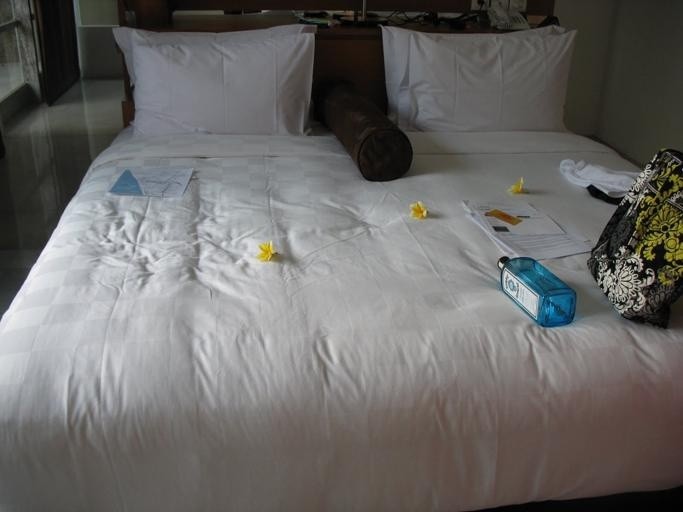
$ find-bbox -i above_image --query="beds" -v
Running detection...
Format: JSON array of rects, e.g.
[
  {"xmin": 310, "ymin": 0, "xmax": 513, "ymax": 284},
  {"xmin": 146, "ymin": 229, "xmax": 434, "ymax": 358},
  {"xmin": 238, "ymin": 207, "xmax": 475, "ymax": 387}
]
[{"xmin": 1, "ymin": 0, "xmax": 683, "ymax": 511}]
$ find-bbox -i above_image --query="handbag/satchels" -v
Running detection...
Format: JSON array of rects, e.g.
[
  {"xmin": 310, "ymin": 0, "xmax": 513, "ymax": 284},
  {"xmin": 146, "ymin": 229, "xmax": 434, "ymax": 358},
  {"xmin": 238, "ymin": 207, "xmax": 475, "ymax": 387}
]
[{"xmin": 586, "ymin": 148, "xmax": 681, "ymax": 328}]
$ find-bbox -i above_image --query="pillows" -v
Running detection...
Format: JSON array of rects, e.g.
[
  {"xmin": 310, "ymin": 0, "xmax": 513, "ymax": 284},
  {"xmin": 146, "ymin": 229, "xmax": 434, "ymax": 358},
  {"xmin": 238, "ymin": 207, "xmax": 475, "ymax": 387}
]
[
  {"xmin": 377, "ymin": 22, "xmax": 578, "ymax": 132},
  {"xmin": 111, "ymin": 23, "xmax": 319, "ymax": 137},
  {"xmin": 312, "ymin": 84, "xmax": 413, "ymax": 182}
]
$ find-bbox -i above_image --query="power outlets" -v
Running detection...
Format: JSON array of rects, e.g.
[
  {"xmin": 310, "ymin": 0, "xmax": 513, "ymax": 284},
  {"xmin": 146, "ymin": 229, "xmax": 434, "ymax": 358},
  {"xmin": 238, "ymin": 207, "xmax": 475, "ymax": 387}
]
[{"xmin": 471, "ymin": 0, "xmax": 490, "ymax": 11}]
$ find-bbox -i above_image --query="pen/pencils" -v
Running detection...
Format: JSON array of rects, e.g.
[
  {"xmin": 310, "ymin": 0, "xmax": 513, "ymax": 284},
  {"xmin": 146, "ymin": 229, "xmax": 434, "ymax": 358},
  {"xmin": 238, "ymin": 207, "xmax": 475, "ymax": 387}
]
[{"xmin": 517, "ymin": 216, "xmax": 546, "ymax": 218}]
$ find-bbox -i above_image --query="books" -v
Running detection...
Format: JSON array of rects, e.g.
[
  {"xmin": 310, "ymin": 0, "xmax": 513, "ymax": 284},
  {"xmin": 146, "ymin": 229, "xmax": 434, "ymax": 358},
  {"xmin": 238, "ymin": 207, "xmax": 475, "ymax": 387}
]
[{"xmin": 461, "ymin": 199, "xmax": 593, "ymax": 259}]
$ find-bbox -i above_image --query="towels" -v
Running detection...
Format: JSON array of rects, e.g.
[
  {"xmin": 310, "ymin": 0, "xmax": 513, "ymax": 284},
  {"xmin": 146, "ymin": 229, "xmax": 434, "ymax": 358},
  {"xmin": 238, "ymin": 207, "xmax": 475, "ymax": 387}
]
[{"xmin": 560, "ymin": 154, "xmax": 642, "ymax": 198}]
